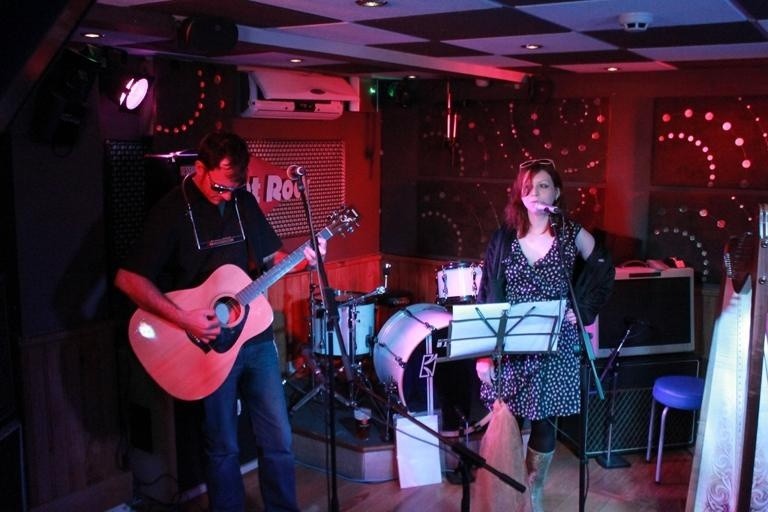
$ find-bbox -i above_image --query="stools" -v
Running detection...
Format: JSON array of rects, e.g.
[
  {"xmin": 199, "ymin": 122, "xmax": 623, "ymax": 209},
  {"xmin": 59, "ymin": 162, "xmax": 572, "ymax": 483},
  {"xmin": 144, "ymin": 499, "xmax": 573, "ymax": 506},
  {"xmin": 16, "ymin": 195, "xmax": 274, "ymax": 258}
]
[{"xmin": 644, "ymin": 374, "xmax": 704, "ymax": 482}]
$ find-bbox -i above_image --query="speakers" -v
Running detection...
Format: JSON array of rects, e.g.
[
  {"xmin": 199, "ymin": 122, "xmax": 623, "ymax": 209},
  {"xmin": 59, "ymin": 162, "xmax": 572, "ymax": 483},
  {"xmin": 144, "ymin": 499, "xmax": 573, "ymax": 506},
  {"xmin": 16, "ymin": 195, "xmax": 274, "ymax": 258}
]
[
  {"xmin": 555, "ymin": 355, "xmax": 699, "ymax": 458},
  {"xmin": 584, "ymin": 260, "xmax": 695, "ymax": 360}
]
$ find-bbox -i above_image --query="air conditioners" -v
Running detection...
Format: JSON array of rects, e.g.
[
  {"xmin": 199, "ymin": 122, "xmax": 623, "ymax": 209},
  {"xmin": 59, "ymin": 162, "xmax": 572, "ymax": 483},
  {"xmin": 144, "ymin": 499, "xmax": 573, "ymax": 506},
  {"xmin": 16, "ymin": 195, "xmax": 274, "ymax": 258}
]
[{"xmin": 239, "ymin": 65, "xmax": 361, "ymax": 121}]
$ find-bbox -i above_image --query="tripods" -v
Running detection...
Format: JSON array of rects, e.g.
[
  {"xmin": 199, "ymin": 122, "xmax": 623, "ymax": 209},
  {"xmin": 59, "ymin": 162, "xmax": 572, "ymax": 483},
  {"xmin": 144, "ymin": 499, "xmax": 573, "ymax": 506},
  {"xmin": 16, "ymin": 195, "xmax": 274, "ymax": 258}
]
[{"xmin": 290, "ymin": 270, "xmax": 395, "ymax": 441}]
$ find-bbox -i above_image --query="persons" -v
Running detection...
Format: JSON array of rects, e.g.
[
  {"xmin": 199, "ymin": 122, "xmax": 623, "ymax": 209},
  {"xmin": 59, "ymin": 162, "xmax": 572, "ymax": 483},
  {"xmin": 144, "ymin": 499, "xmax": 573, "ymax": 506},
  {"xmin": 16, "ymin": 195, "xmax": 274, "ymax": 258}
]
[
  {"xmin": 112, "ymin": 124, "xmax": 327, "ymax": 512},
  {"xmin": 469, "ymin": 157, "xmax": 618, "ymax": 512}
]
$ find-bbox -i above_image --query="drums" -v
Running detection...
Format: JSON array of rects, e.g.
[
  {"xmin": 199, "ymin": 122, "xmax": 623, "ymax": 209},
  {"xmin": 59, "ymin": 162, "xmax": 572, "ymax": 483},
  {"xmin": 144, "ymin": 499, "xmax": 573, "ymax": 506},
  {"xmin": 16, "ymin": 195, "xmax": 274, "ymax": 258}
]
[
  {"xmin": 308, "ymin": 290, "xmax": 379, "ymax": 360},
  {"xmin": 372, "ymin": 303, "xmax": 494, "ymax": 438},
  {"xmin": 435, "ymin": 260, "xmax": 485, "ymax": 305}
]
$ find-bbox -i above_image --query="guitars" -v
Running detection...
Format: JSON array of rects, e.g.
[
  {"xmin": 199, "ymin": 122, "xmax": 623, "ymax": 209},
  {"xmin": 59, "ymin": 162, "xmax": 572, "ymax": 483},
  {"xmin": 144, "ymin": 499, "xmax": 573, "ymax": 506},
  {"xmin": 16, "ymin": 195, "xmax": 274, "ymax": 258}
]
[{"xmin": 127, "ymin": 205, "xmax": 362, "ymax": 401}]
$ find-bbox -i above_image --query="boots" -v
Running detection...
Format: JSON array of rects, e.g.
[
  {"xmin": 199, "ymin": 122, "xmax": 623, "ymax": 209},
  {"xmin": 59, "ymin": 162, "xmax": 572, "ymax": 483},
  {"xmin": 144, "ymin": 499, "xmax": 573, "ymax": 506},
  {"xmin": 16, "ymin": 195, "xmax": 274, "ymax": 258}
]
[{"xmin": 522, "ymin": 444, "xmax": 558, "ymax": 511}]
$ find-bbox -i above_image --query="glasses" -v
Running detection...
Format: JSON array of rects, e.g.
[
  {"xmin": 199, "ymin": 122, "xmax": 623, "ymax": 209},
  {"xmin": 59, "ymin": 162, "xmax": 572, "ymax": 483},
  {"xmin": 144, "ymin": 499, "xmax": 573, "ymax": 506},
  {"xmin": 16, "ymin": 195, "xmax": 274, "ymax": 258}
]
[
  {"xmin": 206, "ymin": 170, "xmax": 247, "ymax": 195},
  {"xmin": 519, "ymin": 159, "xmax": 556, "ymax": 173}
]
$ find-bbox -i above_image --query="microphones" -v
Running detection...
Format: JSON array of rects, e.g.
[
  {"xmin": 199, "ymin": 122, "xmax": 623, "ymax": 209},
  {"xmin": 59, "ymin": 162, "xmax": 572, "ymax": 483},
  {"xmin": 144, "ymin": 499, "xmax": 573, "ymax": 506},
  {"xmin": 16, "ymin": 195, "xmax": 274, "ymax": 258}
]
[
  {"xmin": 536, "ymin": 202, "xmax": 558, "ymax": 214},
  {"xmin": 287, "ymin": 165, "xmax": 305, "ymax": 180}
]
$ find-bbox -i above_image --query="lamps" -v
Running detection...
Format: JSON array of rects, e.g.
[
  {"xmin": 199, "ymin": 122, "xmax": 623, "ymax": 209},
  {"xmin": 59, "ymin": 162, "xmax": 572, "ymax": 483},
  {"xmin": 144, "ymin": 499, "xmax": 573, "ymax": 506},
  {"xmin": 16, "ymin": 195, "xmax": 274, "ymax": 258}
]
[{"xmin": 65, "ymin": 47, "xmax": 154, "ymax": 115}]
